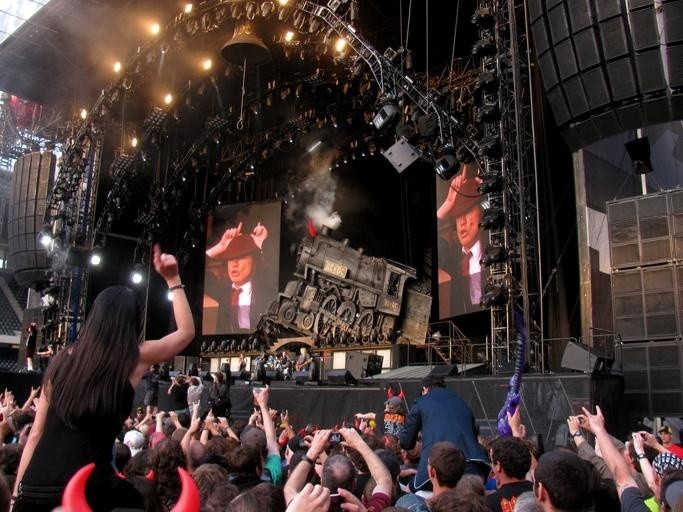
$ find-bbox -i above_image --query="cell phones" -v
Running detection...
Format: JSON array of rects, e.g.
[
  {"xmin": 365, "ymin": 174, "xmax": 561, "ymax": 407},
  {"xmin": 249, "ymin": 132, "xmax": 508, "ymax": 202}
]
[
  {"xmin": 640, "ymin": 434, "xmax": 646, "ymax": 441},
  {"xmin": 252, "ymin": 381, "xmax": 265, "ymax": 410},
  {"xmin": 331, "ymin": 495, "xmax": 345, "ymax": 511},
  {"xmin": 328, "ymin": 432, "xmax": 342, "ymax": 445}
]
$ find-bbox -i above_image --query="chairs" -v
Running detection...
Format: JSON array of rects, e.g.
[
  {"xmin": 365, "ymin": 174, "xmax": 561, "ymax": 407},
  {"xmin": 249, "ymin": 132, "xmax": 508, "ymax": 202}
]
[{"xmin": 0, "ymin": 274, "xmax": 27, "ymax": 373}]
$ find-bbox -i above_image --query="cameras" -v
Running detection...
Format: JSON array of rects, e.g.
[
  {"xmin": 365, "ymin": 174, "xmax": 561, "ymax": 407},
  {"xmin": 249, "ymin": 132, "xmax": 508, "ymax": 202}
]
[{"xmin": 208, "ymin": 395, "xmax": 232, "ymax": 418}]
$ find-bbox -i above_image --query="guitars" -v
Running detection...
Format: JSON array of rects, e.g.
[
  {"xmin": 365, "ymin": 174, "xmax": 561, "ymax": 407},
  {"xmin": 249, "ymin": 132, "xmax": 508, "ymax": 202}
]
[{"xmin": 295, "ymin": 357, "xmax": 314, "ymax": 372}]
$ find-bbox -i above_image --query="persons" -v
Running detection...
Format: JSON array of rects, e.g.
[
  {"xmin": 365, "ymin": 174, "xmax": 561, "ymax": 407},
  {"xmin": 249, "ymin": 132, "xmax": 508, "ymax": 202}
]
[
  {"xmin": 6, "ymin": 245, "xmax": 196, "ymax": 511},
  {"xmin": 204, "ymin": 220, "xmax": 278, "ymax": 335},
  {"xmin": 434, "ymin": 163, "xmax": 495, "ymax": 318},
  {"xmin": 0, "ymin": 344, "xmax": 682, "ymax": 512}
]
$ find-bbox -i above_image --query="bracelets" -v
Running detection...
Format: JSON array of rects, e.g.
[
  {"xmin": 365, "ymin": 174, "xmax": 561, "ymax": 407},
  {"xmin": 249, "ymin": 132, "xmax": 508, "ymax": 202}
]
[{"xmin": 168, "ymin": 284, "xmax": 187, "ymax": 294}]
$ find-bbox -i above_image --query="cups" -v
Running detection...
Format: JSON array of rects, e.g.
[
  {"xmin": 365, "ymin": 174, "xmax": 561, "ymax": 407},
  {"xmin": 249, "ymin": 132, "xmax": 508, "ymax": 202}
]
[{"xmin": 253, "ymin": 386, "xmax": 264, "ymax": 405}]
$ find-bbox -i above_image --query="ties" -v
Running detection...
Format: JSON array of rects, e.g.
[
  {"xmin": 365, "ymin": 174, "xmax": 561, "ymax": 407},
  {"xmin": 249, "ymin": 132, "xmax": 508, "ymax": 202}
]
[
  {"xmin": 230, "ymin": 288, "xmax": 242, "ymax": 329},
  {"xmin": 462, "ymin": 252, "xmax": 472, "ymax": 280}
]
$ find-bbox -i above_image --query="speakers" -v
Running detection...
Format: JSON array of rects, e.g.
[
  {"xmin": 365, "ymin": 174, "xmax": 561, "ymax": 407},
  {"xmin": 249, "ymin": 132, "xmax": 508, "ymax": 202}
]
[
  {"xmin": 610, "ymin": 263, "xmax": 679, "ymax": 344},
  {"xmin": 231, "ymin": 371, "xmax": 243, "ymax": 385},
  {"xmin": 266, "ymin": 371, "xmax": 281, "ymax": 384},
  {"xmin": 616, "ymin": 341, "xmax": 682, "ymax": 417},
  {"xmin": 669, "ymin": 188, "xmax": 683, "ymax": 263},
  {"xmin": 327, "ymin": 370, "xmax": 356, "ymax": 385},
  {"xmin": 430, "ymin": 365, "xmax": 458, "ymax": 377},
  {"xmin": 292, "ymin": 371, "xmax": 313, "ymax": 385},
  {"xmin": 674, "ymin": 262, "xmax": 683, "ymax": 340},
  {"xmin": 200, "ymin": 371, "xmax": 214, "ymax": 381},
  {"xmin": 605, "ymin": 193, "xmax": 674, "ymax": 270}
]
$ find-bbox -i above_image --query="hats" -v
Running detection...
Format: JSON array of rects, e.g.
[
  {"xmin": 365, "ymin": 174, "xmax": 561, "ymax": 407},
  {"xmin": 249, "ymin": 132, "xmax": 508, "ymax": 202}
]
[
  {"xmin": 659, "ymin": 426, "xmax": 672, "ymax": 433},
  {"xmin": 448, "ymin": 181, "xmax": 484, "ymax": 216},
  {"xmin": 222, "ymin": 237, "xmax": 259, "ymax": 259},
  {"xmin": 124, "ymin": 431, "xmax": 145, "ymax": 458}
]
